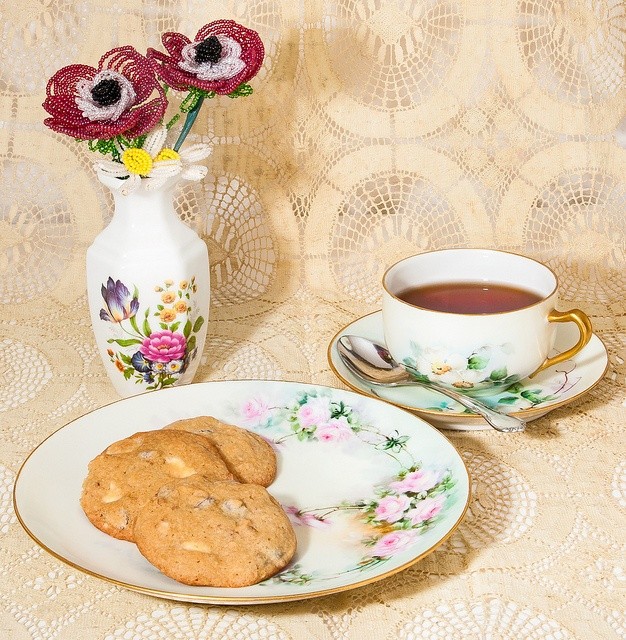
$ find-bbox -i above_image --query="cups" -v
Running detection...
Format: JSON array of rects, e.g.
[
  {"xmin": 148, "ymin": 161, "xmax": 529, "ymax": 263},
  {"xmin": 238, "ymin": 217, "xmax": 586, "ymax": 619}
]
[{"xmin": 381, "ymin": 247, "xmax": 594, "ymax": 390}]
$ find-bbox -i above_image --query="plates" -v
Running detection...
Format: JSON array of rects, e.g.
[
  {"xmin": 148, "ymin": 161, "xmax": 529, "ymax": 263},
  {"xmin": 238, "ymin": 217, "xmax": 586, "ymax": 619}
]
[
  {"xmin": 13, "ymin": 380, "xmax": 473, "ymax": 605},
  {"xmin": 328, "ymin": 308, "xmax": 611, "ymax": 430}
]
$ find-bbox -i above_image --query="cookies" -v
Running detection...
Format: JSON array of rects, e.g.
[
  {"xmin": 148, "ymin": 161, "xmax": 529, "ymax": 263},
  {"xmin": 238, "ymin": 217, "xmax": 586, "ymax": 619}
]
[
  {"xmin": 134, "ymin": 476, "xmax": 296, "ymax": 587},
  {"xmin": 83, "ymin": 431, "xmax": 243, "ymax": 543},
  {"xmin": 160, "ymin": 416, "xmax": 277, "ymax": 487}
]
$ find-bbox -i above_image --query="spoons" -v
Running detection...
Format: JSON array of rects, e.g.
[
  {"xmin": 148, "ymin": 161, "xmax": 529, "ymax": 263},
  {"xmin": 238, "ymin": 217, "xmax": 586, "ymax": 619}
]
[{"xmin": 336, "ymin": 335, "xmax": 527, "ymax": 434}]
